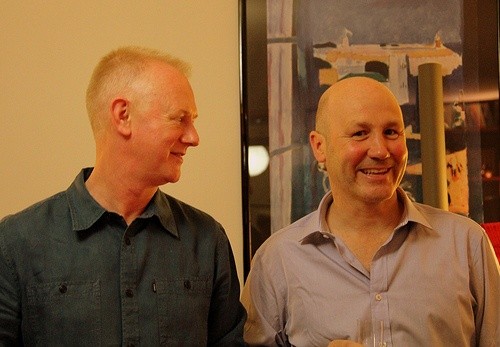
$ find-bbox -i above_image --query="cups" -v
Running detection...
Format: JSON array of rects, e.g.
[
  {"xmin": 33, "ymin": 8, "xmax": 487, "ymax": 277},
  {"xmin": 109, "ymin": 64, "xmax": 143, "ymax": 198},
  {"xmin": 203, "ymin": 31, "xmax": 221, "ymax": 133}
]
[{"xmin": 356, "ymin": 319, "xmax": 384, "ymax": 347}]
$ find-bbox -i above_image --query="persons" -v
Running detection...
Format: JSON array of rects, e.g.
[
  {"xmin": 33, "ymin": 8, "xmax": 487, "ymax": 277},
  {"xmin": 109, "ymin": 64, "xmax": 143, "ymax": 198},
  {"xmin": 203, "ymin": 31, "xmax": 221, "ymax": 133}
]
[
  {"xmin": 243, "ymin": 77, "xmax": 500, "ymax": 347},
  {"xmin": 0, "ymin": 47, "xmax": 247, "ymax": 347}
]
